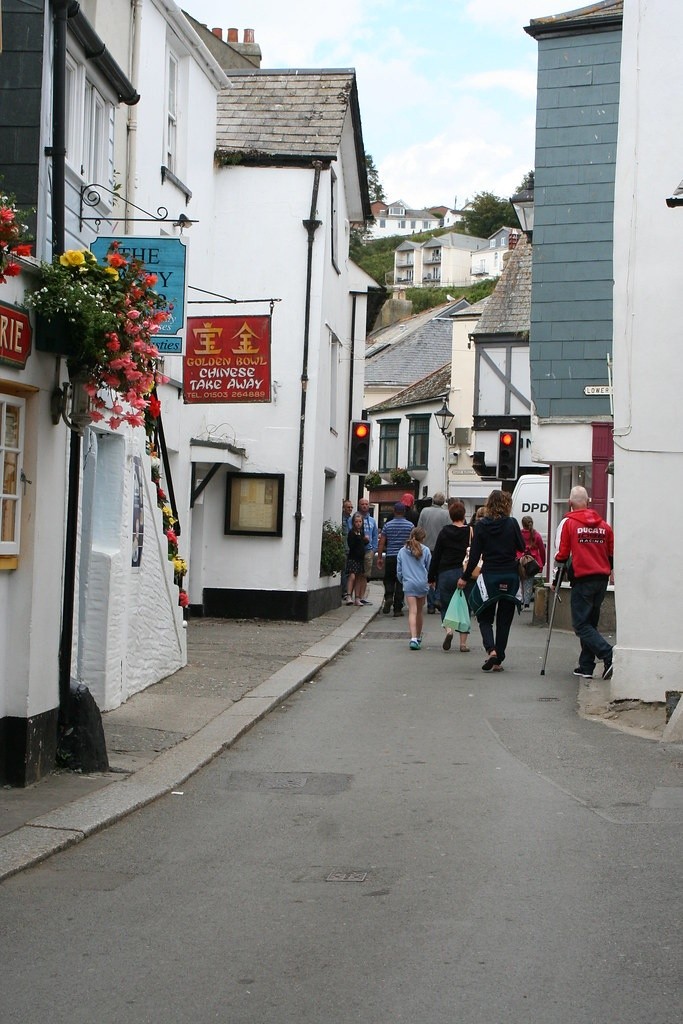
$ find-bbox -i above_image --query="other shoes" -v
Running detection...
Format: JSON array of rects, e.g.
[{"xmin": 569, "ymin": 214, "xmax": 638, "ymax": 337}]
[
  {"xmin": 602, "ymin": 646, "xmax": 613, "ymax": 679},
  {"xmin": 427, "ymin": 608, "xmax": 435, "ymax": 614},
  {"xmin": 354, "ymin": 601, "xmax": 363, "ymax": 606},
  {"xmin": 573, "ymin": 667, "xmax": 593, "ymax": 678},
  {"xmin": 383, "ymin": 598, "xmax": 393, "ymax": 613},
  {"xmin": 408, "ymin": 640, "xmax": 421, "ymax": 649},
  {"xmin": 346, "ymin": 601, "xmax": 352, "ymax": 606},
  {"xmin": 460, "ymin": 646, "xmax": 469, "ymax": 652},
  {"xmin": 443, "ymin": 632, "xmax": 453, "ymax": 650},
  {"xmin": 360, "ymin": 599, "xmax": 371, "ymax": 605},
  {"xmin": 484, "ymin": 659, "xmax": 503, "ymax": 671},
  {"xmin": 393, "ymin": 611, "xmax": 404, "ymax": 617},
  {"xmin": 481, "ymin": 655, "xmax": 500, "ymax": 670}
]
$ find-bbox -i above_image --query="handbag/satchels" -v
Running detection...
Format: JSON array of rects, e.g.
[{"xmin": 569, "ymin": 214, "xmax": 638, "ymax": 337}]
[{"xmin": 441, "ymin": 587, "xmax": 470, "ymax": 633}]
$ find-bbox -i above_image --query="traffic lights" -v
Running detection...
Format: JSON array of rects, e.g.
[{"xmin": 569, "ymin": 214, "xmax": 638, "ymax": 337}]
[
  {"xmin": 494, "ymin": 428, "xmax": 520, "ymax": 481},
  {"xmin": 348, "ymin": 420, "xmax": 372, "ymax": 476}
]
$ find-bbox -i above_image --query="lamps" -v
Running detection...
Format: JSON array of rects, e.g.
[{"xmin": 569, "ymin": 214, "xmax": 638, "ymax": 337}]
[
  {"xmin": 173, "ymin": 214, "xmax": 193, "ymax": 229},
  {"xmin": 434, "ymin": 398, "xmax": 455, "ymax": 440}
]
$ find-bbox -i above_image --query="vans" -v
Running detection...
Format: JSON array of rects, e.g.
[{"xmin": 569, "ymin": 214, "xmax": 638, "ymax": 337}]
[{"xmin": 509, "ymin": 474, "xmax": 549, "ymax": 550}]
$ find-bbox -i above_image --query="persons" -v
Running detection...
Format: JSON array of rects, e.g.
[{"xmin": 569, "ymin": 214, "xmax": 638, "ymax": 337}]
[
  {"xmin": 375, "ymin": 490, "xmax": 485, "ymax": 615},
  {"xmin": 554, "ymin": 486, "xmax": 616, "ymax": 679},
  {"xmin": 456, "ymin": 490, "xmax": 528, "ymax": 672},
  {"xmin": 428, "ymin": 501, "xmax": 471, "ymax": 651},
  {"xmin": 516, "ymin": 515, "xmax": 546, "ymax": 612},
  {"xmin": 343, "ymin": 496, "xmax": 379, "ymax": 605},
  {"xmin": 395, "ymin": 526, "xmax": 431, "ymax": 651}
]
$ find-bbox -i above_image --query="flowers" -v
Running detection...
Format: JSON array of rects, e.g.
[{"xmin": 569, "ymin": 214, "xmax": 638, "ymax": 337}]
[
  {"xmin": 0, "ymin": 173, "xmax": 34, "ymax": 284},
  {"xmin": 21, "ymin": 241, "xmax": 189, "ymax": 610}
]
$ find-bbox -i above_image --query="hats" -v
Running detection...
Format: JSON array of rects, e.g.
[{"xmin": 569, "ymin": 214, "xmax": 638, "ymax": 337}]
[{"xmin": 394, "ymin": 502, "xmax": 405, "ymax": 512}]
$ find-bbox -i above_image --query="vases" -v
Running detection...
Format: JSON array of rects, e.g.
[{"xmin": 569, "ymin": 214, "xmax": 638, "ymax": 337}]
[{"xmin": 34, "ymin": 309, "xmax": 86, "ymax": 359}]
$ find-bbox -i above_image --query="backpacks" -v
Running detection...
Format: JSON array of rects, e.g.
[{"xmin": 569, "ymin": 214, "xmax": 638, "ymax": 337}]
[{"xmin": 518, "ymin": 551, "xmax": 540, "ymax": 578}]
